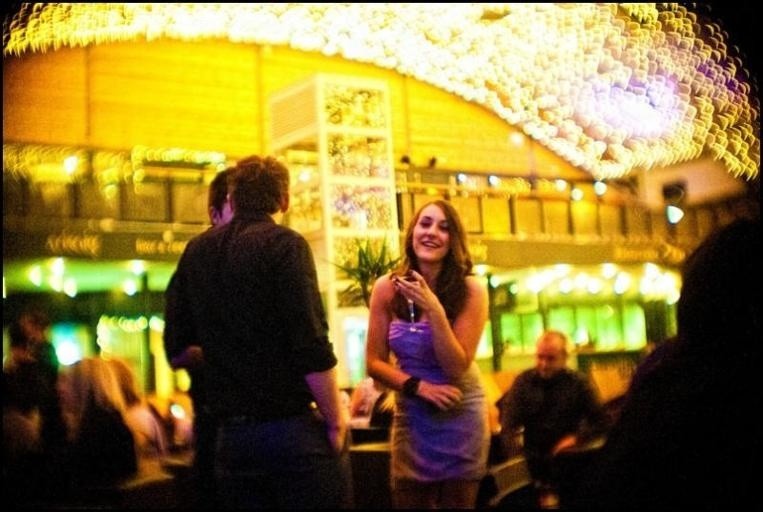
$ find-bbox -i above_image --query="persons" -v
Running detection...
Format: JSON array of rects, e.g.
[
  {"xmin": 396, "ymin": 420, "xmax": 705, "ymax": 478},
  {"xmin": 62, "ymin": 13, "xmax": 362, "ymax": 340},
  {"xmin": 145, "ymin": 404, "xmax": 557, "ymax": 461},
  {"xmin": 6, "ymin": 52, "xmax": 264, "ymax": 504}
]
[
  {"xmin": 179, "ymin": 154, "xmax": 352, "ymax": 511},
  {"xmin": 158, "ymin": 167, "xmax": 241, "ymax": 512},
  {"xmin": 497, "ymin": 215, "xmax": 763, "ymax": 511},
  {"xmin": 0, "ymin": 296, "xmax": 171, "ymax": 510},
  {"xmin": 355, "ymin": 371, "xmax": 394, "ymax": 426},
  {"xmin": 365, "ymin": 199, "xmax": 494, "ymax": 510}
]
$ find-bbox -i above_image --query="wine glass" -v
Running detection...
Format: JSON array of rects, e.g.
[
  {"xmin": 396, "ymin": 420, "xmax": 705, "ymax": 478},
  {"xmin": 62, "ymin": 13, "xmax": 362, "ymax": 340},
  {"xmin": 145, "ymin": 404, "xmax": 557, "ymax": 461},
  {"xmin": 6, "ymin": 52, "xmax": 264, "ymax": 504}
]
[{"xmin": 400, "ymin": 275, "xmax": 424, "ymax": 334}]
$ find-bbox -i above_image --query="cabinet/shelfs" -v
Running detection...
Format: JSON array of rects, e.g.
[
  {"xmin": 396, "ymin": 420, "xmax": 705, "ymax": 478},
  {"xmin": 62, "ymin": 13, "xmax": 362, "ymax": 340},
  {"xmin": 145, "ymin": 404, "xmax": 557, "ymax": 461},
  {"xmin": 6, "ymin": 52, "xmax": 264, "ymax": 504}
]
[{"xmin": 264, "ymin": 77, "xmax": 404, "ymax": 389}]
